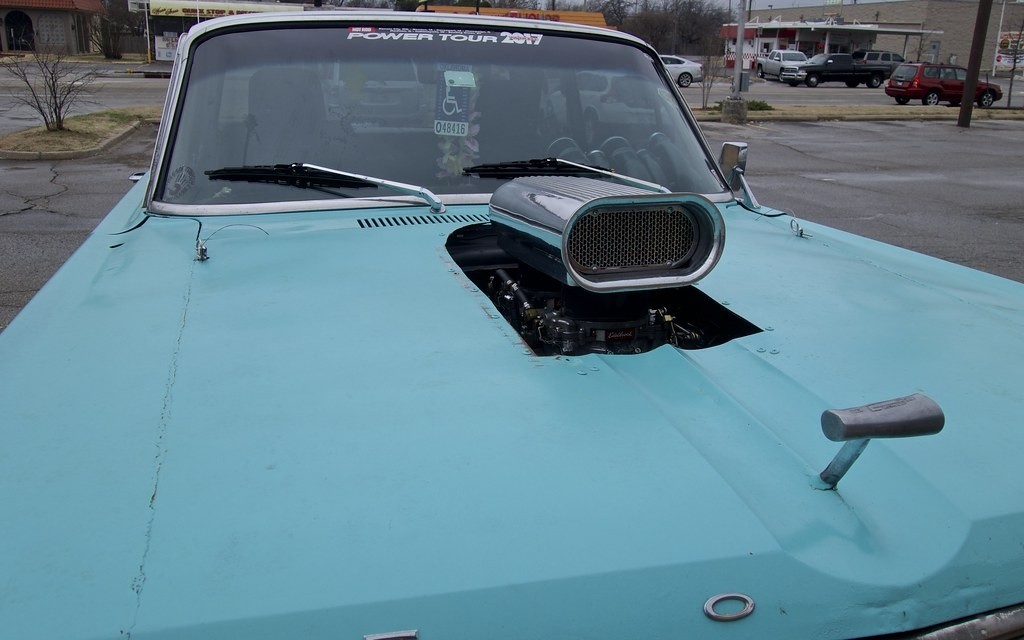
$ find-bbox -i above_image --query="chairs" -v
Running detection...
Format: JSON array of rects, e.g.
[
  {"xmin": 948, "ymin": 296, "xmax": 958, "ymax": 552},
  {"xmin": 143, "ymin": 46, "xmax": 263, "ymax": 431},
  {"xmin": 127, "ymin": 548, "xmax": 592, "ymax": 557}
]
[
  {"xmin": 465, "ymin": 81, "xmax": 574, "ymax": 176},
  {"xmin": 191, "ymin": 69, "xmax": 372, "ymax": 185}
]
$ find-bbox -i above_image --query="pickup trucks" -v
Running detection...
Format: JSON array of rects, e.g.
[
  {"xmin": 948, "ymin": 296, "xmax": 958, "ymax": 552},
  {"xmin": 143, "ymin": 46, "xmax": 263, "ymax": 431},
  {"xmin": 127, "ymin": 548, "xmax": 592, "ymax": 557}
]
[
  {"xmin": 755, "ymin": 49, "xmax": 809, "ymax": 84},
  {"xmin": 779, "ymin": 53, "xmax": 892, "ymax": 89}
]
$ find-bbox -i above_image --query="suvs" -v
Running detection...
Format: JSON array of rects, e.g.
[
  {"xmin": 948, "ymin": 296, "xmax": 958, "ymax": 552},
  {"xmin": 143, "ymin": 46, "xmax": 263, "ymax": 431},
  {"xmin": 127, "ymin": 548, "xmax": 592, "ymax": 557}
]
[
  {"xmin": 852, "ymin": 48, "xmax": 905, "ymax": 80},
  {"xmin": 883, "ymin": 61, "xmax": 1004, "ymax": 109}
]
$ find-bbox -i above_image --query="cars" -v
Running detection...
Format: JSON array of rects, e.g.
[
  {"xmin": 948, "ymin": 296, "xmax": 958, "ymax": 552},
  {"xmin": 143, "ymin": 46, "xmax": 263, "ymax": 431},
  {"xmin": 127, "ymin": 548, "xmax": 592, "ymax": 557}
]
[
  {"xmin": 1, "ymin": 10, "xmax": 1024, "ymax": 639},
  {"xmin": 660, "ymin": 54, "xmax": 706, "ymax": 88}
]
